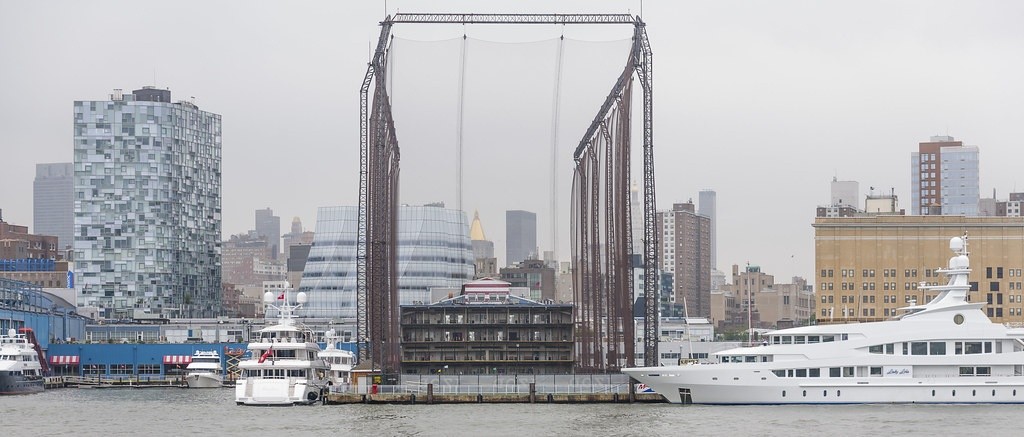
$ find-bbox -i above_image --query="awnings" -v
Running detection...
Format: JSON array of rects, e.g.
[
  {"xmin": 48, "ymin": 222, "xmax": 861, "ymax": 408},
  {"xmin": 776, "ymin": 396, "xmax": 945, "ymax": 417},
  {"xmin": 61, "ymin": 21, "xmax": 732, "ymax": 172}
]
[
  {"xmin": 50, "ymin": 356, "xmax": 80, "ymax": 364},
  {"xmin": 163, "ymin": 355, "xmax": 192, "ymax": 364}
]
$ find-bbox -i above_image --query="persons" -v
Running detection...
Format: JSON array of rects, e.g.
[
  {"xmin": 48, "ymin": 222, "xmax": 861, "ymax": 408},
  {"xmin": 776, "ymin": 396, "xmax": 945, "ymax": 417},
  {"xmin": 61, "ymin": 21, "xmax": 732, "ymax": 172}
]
[{"xmin": 762, "ymin": 337, "xmax": 766, "ymax": 342}]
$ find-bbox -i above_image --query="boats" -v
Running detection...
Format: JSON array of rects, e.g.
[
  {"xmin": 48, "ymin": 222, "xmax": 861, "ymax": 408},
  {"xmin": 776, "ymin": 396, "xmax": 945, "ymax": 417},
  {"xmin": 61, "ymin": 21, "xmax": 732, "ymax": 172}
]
[
  {"xmin": 620, "ymin": 230, "xmax": 1024, "ymax": 404},
  {"xmin": 185, "ymin": 350, "xmax": 223, "ymax": 387},
  {"xmin": 319, "ymin": 328, "xmax": 355, "ymax": 393},
  {"xmin": 234, "ymin": 277, "xmax": 331, "ymax": 406},
  {"xmin": 0, "ymin": 329, "xmax": 45, "ymax": 395}
]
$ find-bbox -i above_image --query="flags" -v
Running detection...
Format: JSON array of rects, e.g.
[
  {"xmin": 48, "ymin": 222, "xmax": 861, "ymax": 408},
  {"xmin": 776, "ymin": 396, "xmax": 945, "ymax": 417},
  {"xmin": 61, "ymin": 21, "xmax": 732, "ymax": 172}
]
[
  {"xmin": 278, "ymin": 294, "xmax": 284, "ymax": 300},
  {"xmin": 258, "ymin": 347, "xmax": 272, "ymax": 363}
]
